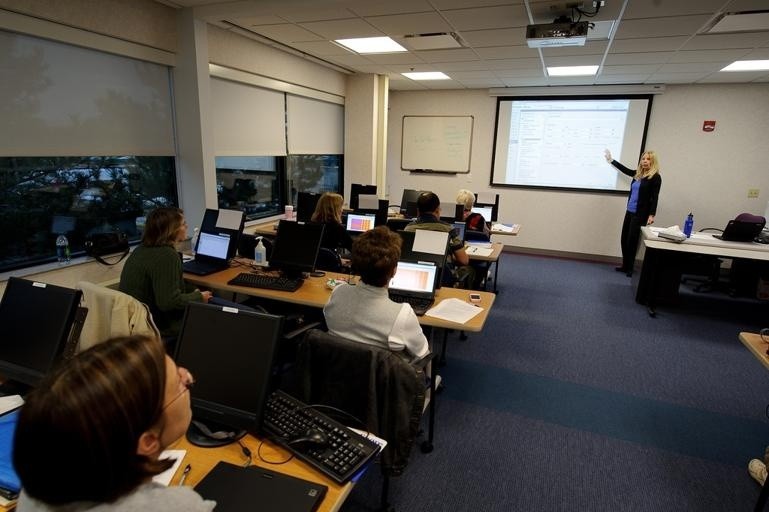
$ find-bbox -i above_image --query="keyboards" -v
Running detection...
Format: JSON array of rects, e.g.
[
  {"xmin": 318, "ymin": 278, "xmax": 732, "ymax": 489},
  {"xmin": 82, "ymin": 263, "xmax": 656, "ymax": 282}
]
[
  {"xmin": 253, "ymin": 387, "xmax": 382, "ymax": 485},
  {"xmin": 226, "ymin": 271, "xmax": 305, "ymax": 292}
]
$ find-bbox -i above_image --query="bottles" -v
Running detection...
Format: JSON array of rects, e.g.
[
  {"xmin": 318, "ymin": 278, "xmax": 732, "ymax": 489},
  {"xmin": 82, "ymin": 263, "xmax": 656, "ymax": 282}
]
[
  {"xmin": 285, "ymin": 205, "xmax": 294, "ymax": 220},
  {"xmin": 55, "ymin": 232, "xmax": 72, "ymax": 265},
  {"xmin": 682, "ymin": 213, "xmax": 694, "ymax": 238}
]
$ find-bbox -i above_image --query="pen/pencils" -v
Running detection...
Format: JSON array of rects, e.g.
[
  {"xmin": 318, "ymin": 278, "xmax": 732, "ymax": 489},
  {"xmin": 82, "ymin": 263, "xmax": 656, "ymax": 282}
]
[
  {"xmin": 178, "ymin": 464, "xmax": 192, "ymax": 486},
  {"xmin": 473, "ymin": 248, "xmax": 478, "ymax": 252}
]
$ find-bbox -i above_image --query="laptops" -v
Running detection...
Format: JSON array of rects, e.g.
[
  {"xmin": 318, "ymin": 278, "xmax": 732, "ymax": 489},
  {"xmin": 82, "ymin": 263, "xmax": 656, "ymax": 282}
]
[
  {"xmin": 343, "ymin": 211, "xmax": 379, "ymax": 238},
  {"xmin": 49, "ymin": 214, "xmax": 78, "ymax": 235},
  {"xmin": 713, "ymin": 219, "xmax": 763, "ymax": 244},
  {"xmin": 387, "ymin": 259, "xmax": 438, "ymax": 316},
  {"xmin": 448, "ymin": 220, "xmax": 467, "ymax": 242},
  {"xmin": 469, "ymin": 204, "xmax": 494, "ymax": 229},
  {"xmin": 180, "ymin": 230, "xmax": 232, "ymax": 277},
  {"xmin": 192, "ymin": 459, "xmax": 331, "ymax": 511}
]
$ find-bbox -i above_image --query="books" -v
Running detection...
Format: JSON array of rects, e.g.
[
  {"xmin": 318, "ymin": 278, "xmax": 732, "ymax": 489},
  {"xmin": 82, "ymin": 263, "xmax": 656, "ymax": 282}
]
[{"xmin": 658, "ymin": 224, "xmax": 687, "ymax": 241}]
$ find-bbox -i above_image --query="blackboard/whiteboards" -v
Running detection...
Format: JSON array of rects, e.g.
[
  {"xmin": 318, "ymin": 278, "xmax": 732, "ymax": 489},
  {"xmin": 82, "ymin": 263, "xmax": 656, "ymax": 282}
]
[{"xmin": 400, "ymin": 114, "xmax": 474, "ymax": 175}]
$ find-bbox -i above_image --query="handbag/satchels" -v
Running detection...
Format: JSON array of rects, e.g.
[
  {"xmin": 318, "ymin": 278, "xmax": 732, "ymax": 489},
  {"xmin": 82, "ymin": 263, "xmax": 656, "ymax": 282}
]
[{"xmin": 82, "ymin": 233, "xmax": 129, "ymax": 265}]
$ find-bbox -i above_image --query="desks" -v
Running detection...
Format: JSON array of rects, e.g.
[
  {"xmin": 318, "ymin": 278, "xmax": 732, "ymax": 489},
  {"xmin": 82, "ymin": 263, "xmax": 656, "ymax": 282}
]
[
  {"xmin": 739, "ymin": 331, "xmax": 769, "ymax": 511},
  {"xmin": 631, "ymin": 227, "xmax": 769, "ymax": 326}
]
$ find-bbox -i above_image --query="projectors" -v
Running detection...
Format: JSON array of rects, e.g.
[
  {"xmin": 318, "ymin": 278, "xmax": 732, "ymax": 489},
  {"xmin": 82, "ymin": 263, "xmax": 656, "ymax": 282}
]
[{"xmin": 524, "ymin": 20, "xmax": 589, "ymax": 49}]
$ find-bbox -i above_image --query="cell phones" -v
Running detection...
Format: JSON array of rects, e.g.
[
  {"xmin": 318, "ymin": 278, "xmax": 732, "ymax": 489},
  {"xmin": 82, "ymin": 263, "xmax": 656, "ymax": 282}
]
[{"xmin": 469, "ymin": 293, "xmax": 483, "ymax": 304}]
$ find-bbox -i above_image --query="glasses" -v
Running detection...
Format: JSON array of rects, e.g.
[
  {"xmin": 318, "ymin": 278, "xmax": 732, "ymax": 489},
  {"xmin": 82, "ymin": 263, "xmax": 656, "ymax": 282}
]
[{"xmin": 161, "ymin": 366, "xmax": 195, "ymax": 411}]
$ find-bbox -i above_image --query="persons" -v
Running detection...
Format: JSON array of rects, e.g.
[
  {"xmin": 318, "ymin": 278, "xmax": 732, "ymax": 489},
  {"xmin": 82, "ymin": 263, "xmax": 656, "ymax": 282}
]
[
  {"xmin": 605, "ymin": 150, "xmax": 662, "ymax": 278},
  {"xmin": 302, "ymin": 190, "xmax": 355, "ymax": 275},
  {"xmin": 323, "ymin": 227, "xmax": 430, "ymax": 368},
  {"xmin": 453, "ymin": 188, "xmax": 495, "ymax": 241},
  {"xmin": 403, "ymin": 191, "xmax": 474, "ymax": 288},
  {"xmin": 12, "ymin": 333, "xmax": 217, "ymax": 512},
  {"xmin": 118, "ymin": 204, "xmax": 213, "ymax": 358}
]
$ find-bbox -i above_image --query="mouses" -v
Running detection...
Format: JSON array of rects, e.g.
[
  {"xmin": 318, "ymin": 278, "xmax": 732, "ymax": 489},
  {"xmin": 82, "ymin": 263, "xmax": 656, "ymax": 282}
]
[
  {"xmin": 287, "ymin": 428, "xmax": 328, "ymax": 451},
  {"xmin": 289, "ymin": 428, "xmax": 329, "ymax": 449}
]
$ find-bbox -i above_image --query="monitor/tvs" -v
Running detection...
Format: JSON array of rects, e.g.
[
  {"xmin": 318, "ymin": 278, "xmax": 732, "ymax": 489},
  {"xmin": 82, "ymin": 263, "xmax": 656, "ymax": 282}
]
[
  {"xmin": 172, "ymin": 301, "xmax": 286, "ymax": 449},
  {"xmin": 395, "ymin": 229, "xmax": 452, "ymax": 289},
  {"xmin": 398, "ymin": 187, "xmax": 432, "ymax": 218},
  {"xmin": 0, "ymin": 276, "xmax": 84, "ymax": 391},
  {"xmin": 268, "ymin": 219, "xmax": 325, "ymax": 277},
  {"xmin": 473, "ymin": 192, "xmax": 499, "ymax": 222},
  {"xmin": 347, "ymin": 183, "xmax": 379, "ymax": 210},
  {"xmin": 193, "ymin": 207, "xmax": 247, "ymax": 260},
  {"xmin": 296, "ymin": 191, "xmax": 323, "ymax": 222},
  {"xmin": 353, "ymin": 195, "xmax": 390, "ymax": 226}
]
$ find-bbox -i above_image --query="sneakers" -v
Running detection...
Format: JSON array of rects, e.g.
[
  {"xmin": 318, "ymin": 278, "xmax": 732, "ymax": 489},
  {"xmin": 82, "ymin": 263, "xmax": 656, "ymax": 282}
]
[
  {"xmin": 615, "ymin": 265, "xmax": 633, "ymax": 277},
  {"xmin": 748, "ymin": 459, "xmax": 768, "ymax": 487}
]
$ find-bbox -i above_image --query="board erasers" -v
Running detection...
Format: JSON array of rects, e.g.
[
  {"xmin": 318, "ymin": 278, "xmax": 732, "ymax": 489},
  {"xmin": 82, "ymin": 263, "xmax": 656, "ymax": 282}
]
[{"xmin": 414, "ymin": 169, "xmax": 433, "ymax": 173}]
